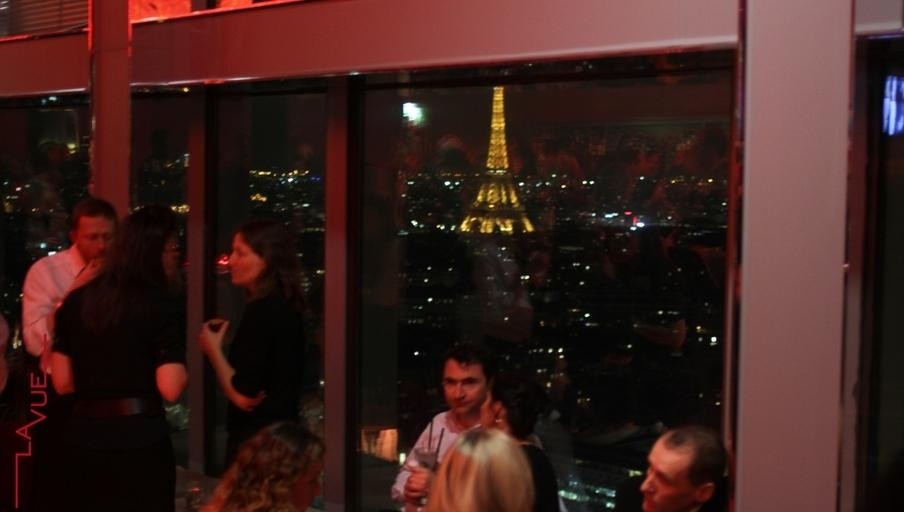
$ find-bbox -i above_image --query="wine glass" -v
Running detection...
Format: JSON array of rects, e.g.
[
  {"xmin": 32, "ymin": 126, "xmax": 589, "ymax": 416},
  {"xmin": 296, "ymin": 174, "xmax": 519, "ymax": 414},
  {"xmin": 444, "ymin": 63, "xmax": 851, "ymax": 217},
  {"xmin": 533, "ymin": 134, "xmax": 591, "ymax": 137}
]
[{"xmin": 414, "ymin": 448, "xmax": 438, "ymax": 505}]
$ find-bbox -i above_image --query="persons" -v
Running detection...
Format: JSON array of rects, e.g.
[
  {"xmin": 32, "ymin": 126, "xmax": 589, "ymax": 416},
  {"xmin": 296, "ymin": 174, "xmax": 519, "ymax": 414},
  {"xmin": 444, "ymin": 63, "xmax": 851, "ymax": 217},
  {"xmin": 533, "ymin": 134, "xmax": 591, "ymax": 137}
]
[
  {"xmin": 478, "ymin": 362, "xmax": 561, "ymax": 510},
  {"xmin": 387, "ymin": 341, "xmax": 496, "ymax": 511},
  {"xmin": 193, "ymin": 213, "xmax": 303, "ymax": 479},
  {"xmin": 638, "ymin": 426, "xmax": 725, "ymax": 512},
  {"xmin": 44, "ymin": 204, "xmax": 191, "ymax": 510},
  {"xmin": 419, "ymin": 425, "xmax": 536, "ymax": 511},
  {"xmin": 21, "ymin": 197, "xmax": 119, "ymax": 379}
]
